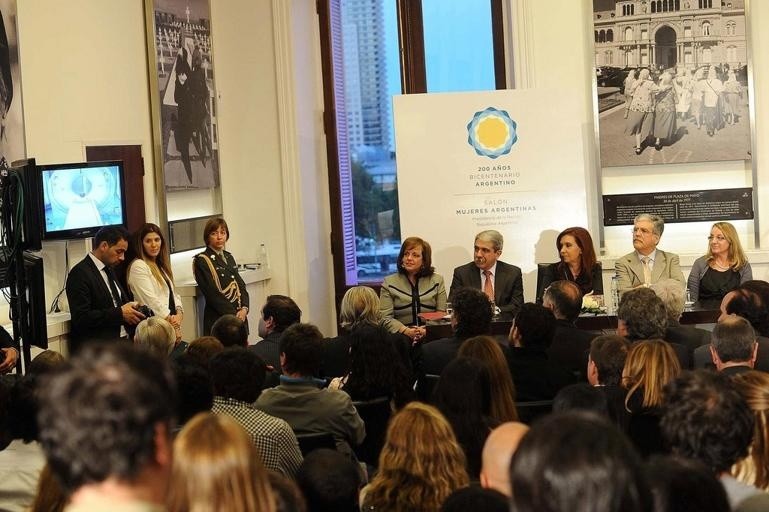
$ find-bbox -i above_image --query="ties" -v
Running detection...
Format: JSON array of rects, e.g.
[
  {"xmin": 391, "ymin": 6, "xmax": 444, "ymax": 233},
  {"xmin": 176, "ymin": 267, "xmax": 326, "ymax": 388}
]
[
  {"xmin": 103, "ymin": 265, "xmax": 123, "ymax": 306},
  {"xmin": 483, "ymin": 271, "xmax": 494, "ymax": 299},
  {"xmin": 641, "ymin": 257, "xmax": 652, "ymax": 284},
  {"xmin": 220, "ymin": 252, "xmax": 227, "ymax": 264}
]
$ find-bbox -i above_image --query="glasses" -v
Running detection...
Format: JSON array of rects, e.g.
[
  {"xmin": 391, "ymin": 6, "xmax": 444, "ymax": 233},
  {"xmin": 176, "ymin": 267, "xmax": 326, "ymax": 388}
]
[
  {"xmin": 708, "ymin": 235, "xmax": 726, "ymax": 241},
  {"xmin": 632, "ymin": 228, "xmax": 652, "ymax": 236}
]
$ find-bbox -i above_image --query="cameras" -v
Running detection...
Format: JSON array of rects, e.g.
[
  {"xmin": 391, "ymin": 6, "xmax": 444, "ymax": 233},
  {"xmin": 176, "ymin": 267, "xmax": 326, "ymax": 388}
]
[{"xmin": 123, "ymin": 305, "xmax": 155, "ymax": 342}]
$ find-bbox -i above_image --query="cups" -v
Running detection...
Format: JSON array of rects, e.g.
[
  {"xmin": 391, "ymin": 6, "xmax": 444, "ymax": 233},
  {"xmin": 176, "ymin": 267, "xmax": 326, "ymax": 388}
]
[
  {"xmin": 685, "ymin": 289, "xmax": 690, "ymax": 303},
  {"xmin": 446, "ymin": 303, "xmax": 453, "ymax": 315}
]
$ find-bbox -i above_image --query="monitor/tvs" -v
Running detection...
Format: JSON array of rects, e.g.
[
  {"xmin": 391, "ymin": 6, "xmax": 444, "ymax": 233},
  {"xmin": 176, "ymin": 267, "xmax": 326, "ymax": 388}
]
[
  {"xmin": 10, "ymin": 158, "xmax": 41, "ymax": 251},
  {"xmin": 36, "ymin": 160, "xmax": 127, "ymax": 239},
  {"xmin": 12, "ymin": 252, "xmax": 47, "ymax": 350}
]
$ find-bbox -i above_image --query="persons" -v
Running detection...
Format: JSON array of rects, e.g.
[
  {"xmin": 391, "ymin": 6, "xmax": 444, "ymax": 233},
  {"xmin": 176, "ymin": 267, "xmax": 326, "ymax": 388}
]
[
  {"xmin": 326, "ymin": 326, "xmax": 405, "ymax": 422},
  {"xmin": 0, "ymin": 325, "xmax": 20, "ymax": 373},
  {"xmin": 323, "ymin": 286, "xmax": 416, "ymax": 378},
  {"xmin": 608, "ymin": 340, "xmax": 682, "ymax": 457},
  {"xmin": 693, "ymin": 286, "xmax": 768, "ymax": 371},
  {"xmin": 479, "ymin": 422, "xmax": 531, "ymax": 512},
  {"xmin": 722, "ymin": 69, "xmax": 743, "ymax": 123},
  {"xmin": 168, "ymin": 354, "xmax": 214, "ymax": 431},
  {"xmin": 192, "ymin": 218, "xmax": 250, "ymax": 340},
  {"xmin": 425, "ymin": 358, "xmax": 497, "ymax": 482},
  {"xmin": 180, "ymin": 336, "xmax": 223, "ymax": 384},
  {"xmin": 163, "ymin": 411, "xmax": 279, "ymax": 511},
  {"xmin": 687, "ymin": 222, "xmax": 752, "ymax": 305},
  {"xmin": 290, "ymin": 450, "xmax": 363, "ymax": 511},
  {"xmin": 741, "ymin": 281, "xmax": 768, "ymax": 304},
  {"xmin": 699, "ymin": 65, "xmax": 724, "ymax": 135},
  {"xmin": 34, "ymin": 351, "xmax": 63, "ymax": 368},
  {"xmin": 1, "ymin": 374, "xmax": 72, "ymax": 512},
  {"xmin": 587, "ymin": 334, "xmax": 631, "ymax": 388},
  {"xmin": 671, "ymin": 66, "xmax": 702, "ymax": 128},
  {"xmin": 66, "ymin": 226, "xmax": 146, "ymax": 349},
  {"xmin": 449, "ymin": 230, "xmax": 524, "ymax": 315},
  {"xmin": 255, "ymin": 296, "xmax": 303, "ymax": 387},
  {"xmin": 618, "ymin": 287, "xmax": 667, "ymax": 341},
  {"xmin": 720, "ymin": 370, "xmax": 769, "ymax": 511},
  {"xmin": 709, "ymin": 316, "xmax": 760, "ymax": 375},
  {"xmin": 500, "ymin": 302, "xmax": 558, "ymax": 403},
  {"xmin": 637, "ymin": 368, "xmax": 769, "ymax": 512},
  {"xmin": 253, "ymin": 323, "xmax": 367, "ymax": 512},
  {"xmin": 615, "ymin": 214, "xmax": 686, "ymax": 300},
  {"xmin": 439, "ymin": 486, "xmax": 511, "ymax": 512},
  {"xmin": 32, "ymin": 343, "xmax": 173, "ymax": 512},
  {"xmin": 509, "ymin": 412, "xmax": 655, "ymax": 512},
  {"xmin": 459, "ymin": 336, "xmax": 518, "ymax": 426},
  {"xmin": 538, "ymin": 226, "xmax": 605, "ymax": 312},
  {"xmin": 653, "ymin": 72, "xmax": 679, "ymax": 150},
  {"xmin": 624, "ymin": 69, "xmax": 658, "ymax": 155},
  {"xmin": 359, "ymin": 403, "xmax": 469, "ymax": 512},
  {"xmin": 623, "ymin": 70, "xmax": 637, "ymax": 119},
  {"xmin": 126, "ymin": 223, "xmax": 183, "ymax": 344},
  {"xmin": 134, "ymin": 316, "xmax": 176, "ymax": 363},
  {"xmin": 212, "ymin": 314, "xmax": 250, "ymax": 348},
  {"xmin": 420, "ymin": 287, "xmax": 515, "ymax": 380},
  {"xmin": 651, "ymin": 278, "xmax": 686, "ymax": 328},
  {"xmin": 378, "ymin": 237, "xmax": 448, "ymax": 347},
  {"xmin": 543, "ymin": 280, "xmax": 604, "ymax": 376},
  {"xmin": 552, "ymin": 384, "xmax": 608, "ymax": 423},
  {"xmin": 213, "ymin": 346, "xmax": 303, "ymax": 487}
]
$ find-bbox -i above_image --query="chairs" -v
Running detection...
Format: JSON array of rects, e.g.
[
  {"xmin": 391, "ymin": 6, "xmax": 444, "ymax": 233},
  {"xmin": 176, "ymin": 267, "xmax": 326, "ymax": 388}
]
[
  {"xmin": 293, "ymin": 429, "xmax": 337, "ymax": 455},
  {"xmin": 424, "ymin": 373, "xmax": 440, "ymax": 394},
  {"xmin": 512, "ymin": 401, "xmax": 554, "ymax": 424},
  {"xmin": 348, "ymin": 395, "xmax": 394, "ymax": 424},
  {"xmin": 537, "ymin": 263, "xmax": 554, "ymax": 300}
]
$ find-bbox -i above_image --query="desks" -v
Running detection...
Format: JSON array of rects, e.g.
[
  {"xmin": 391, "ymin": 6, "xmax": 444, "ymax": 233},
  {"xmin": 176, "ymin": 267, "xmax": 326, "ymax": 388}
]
[{"xmin": 423, "ymin": 302, "xmax": 725, "ymax": 341}]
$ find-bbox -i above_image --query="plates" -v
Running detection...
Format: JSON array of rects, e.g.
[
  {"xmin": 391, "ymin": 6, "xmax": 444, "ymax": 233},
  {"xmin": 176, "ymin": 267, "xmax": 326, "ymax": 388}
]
[
  {"xmin": 685, "ymin": 301, "xmax": 695, "ymax": 308},
  {"xmin": 441, "ymin": 317, "xmax": 452, "ymax": 322}
]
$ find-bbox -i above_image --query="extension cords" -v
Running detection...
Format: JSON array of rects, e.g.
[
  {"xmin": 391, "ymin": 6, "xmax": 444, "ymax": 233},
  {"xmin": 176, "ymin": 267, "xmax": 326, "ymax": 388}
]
[{"xmin": 46, "ymin": 311, "xmax": 70, "ymax": 323}]
